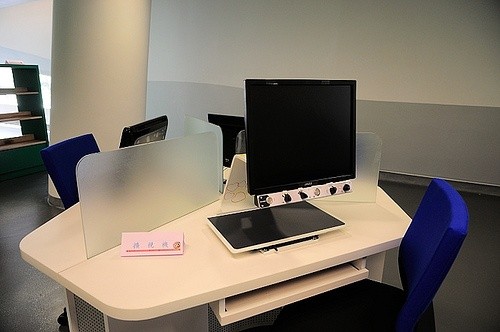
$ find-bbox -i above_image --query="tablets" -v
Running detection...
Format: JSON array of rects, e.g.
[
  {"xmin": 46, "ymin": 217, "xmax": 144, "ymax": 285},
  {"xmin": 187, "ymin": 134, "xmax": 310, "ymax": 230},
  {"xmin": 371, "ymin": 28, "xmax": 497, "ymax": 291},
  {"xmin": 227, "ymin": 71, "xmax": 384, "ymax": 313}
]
[{"xmin": 205, "ymin": 199, "xmax": 347, "ymax": 255}]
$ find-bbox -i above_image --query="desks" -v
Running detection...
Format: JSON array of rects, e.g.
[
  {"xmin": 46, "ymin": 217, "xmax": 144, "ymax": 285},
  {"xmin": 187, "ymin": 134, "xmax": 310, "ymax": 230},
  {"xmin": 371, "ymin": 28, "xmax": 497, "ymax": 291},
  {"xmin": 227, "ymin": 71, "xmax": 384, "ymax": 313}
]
[{"xmin": 19, "ymin": 167, "xmax": 412, "ymax": 332}]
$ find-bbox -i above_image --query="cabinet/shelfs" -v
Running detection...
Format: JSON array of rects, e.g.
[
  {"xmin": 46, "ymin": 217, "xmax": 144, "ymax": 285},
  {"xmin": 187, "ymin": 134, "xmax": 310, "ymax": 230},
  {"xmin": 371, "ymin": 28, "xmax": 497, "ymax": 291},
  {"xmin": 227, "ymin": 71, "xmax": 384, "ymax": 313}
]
[{"xmin": 0, "ymin": 64, "xmax": 48, "ymax": 180}]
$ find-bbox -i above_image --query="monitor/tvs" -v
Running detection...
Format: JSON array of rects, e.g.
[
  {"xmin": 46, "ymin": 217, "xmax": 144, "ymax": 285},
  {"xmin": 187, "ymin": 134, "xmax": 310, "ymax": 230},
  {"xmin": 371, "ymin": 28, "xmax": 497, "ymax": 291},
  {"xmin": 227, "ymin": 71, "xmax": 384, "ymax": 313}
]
[
  {"xmin": 243, "ymin": 79, "xmax": 356, "ymax": 209},
  {"xmin": 208, "ymin": 114, "xmax": 245, "ymax": 168},
  {"xmin": 121, "ymin": 115, "xmax": 168, "ymax": 147}
]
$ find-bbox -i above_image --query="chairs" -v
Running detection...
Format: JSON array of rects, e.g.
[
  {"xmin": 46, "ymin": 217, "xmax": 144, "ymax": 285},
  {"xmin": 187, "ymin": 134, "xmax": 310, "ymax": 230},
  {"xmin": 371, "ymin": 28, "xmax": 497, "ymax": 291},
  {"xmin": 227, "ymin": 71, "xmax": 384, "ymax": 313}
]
[
  {"xmin": 270, "ymin": 178, "xmax": 469, "ymax": 332},
  {"xmin": 40, "ymin": 133, "xmax": 101, "ymax": 326}
]
